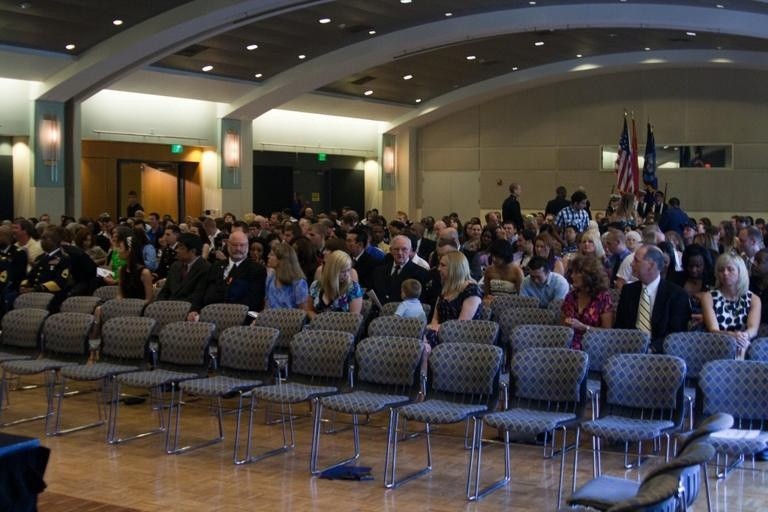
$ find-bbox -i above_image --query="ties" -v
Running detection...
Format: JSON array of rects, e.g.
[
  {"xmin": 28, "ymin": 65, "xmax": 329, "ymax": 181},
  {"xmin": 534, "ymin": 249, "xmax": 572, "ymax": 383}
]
[
  {"xmin": 228, "ymin": 262, "xmax": 237, "ymax": 281},
  {"xmin": 636, "ymin": 287, "xmax": 654, "ymax": 343},
  {"xmin": 391, "ymin": 265, "xmax": 401, "ymax": 277}
]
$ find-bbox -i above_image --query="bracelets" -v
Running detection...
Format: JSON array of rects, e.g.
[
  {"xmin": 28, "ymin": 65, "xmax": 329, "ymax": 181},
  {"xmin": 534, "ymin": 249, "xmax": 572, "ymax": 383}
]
[
  {"xmin": 747, "ymin": 329, "xmax": 753, "ymax": 341},
  {"xmin": 586, "ymin": 324, "xmax": 591, "ymax": 334}
]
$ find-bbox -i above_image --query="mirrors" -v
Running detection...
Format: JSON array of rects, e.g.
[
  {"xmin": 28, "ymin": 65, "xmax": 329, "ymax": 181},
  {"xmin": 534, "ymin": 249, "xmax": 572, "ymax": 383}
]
[{"xmin": 599, "ymin": 143, "xmax": 735, "ymax": 171}]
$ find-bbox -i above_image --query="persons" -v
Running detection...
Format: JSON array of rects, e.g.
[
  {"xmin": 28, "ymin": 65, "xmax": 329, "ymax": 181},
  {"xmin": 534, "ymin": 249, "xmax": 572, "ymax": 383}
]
[
  {"xmin": 463, "ymin": 182, "xmax": 767, "ymax": 323},
  {"xmin": 689, "ymin": 146, "xmax": 705, "ymax": 167},
  {"xmin": 2, "ymin": 190, "xmax": 473, "ymax": 324},
  {"xmin": 614, "ymin": 245, "xmax": 692, "ymax": 358},
  {"xmin": 417, "ymin": 249, "xmax": 483, "ymax": 400},
  {"xmin": 559, "ymin": 254, "xmax": 614, "ymax": 350},
  {"xmin": 700, "ymin": 249, "xmax": 760, "ymax": 362}
]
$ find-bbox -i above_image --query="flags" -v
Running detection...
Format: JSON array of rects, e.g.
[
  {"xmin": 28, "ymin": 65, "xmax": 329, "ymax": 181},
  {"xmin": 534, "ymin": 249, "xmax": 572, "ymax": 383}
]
[
  {"xmin": 628, "ymin": 111, "xmax": 640, "ymax": 195},
  {"xmin": 646, "ymin": 112, "xmax": 651, "ymax": 144},
  {"xmin": 615, "ymin": 108, "xmax": 635, "ymax": 196},
  {"xmin": 643, "ymin": 124, "xmax": 658, "ymax": 190}
]
